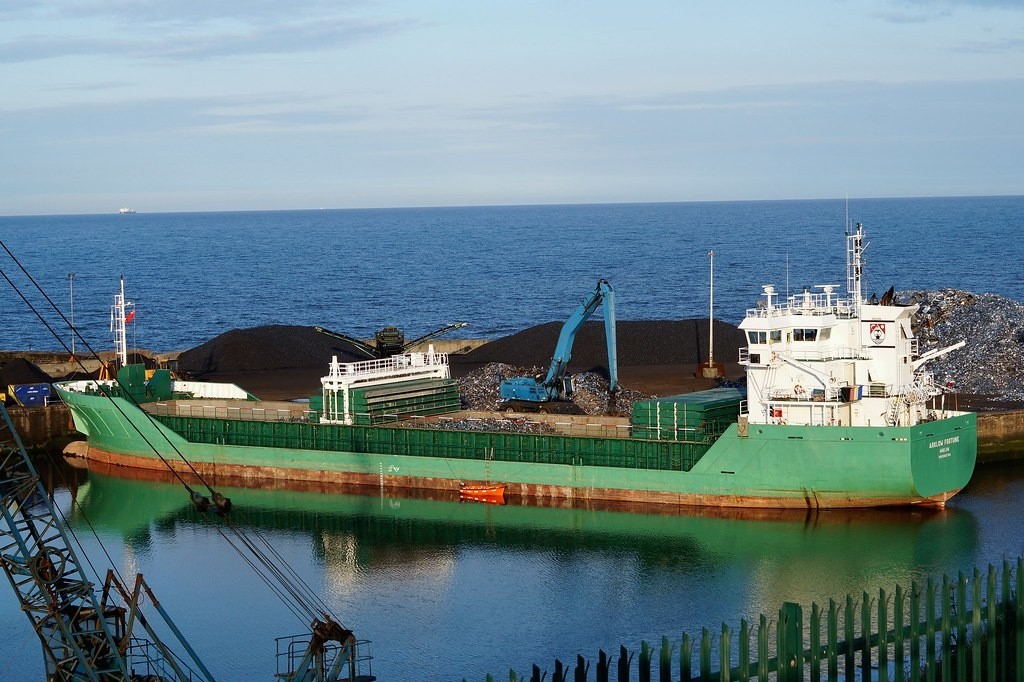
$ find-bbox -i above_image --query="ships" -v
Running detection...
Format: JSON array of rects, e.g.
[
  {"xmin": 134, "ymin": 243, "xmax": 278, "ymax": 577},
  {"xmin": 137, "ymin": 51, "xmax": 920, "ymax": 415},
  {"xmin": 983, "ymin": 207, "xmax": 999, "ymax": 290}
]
[{"xmin": 51, "ymin": 195, "xmax": 979, "ymax": 513}]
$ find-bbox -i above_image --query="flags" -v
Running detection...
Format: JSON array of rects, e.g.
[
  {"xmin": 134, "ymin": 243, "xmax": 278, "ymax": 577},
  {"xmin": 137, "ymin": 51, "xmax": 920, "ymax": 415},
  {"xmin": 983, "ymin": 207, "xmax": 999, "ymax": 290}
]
[{"xmin": 126, "ymin": 309, "xmax": 135, "ymax": 323}]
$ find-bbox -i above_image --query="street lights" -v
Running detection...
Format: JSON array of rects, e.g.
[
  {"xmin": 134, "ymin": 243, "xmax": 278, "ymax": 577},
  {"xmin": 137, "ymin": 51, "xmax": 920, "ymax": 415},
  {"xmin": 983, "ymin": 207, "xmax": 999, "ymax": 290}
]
[
  {"xmin": 707, "ymin": 250, "xmax": 716, "ymax": 369},
  {"xmin": 68, "ymin": 273, "xmax": 76, "ymax": 355}
]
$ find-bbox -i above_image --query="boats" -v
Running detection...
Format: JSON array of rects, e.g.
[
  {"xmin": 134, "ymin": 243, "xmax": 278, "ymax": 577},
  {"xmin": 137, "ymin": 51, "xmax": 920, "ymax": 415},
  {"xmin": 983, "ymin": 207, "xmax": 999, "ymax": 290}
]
[{"xmin": 119, "ymin": 208, "xmax": 136, "ymax": 214}]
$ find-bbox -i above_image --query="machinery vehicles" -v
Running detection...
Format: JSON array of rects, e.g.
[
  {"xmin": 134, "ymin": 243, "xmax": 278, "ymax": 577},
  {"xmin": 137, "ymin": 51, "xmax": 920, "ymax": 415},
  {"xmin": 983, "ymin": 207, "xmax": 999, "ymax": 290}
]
[
  {"xmin": 497, "ymin": 278, "xmax": 621, "ymax": 417},
  {"xmin": 98, "ymin": 360, "xmax": 177, "ymax": 383}
]
